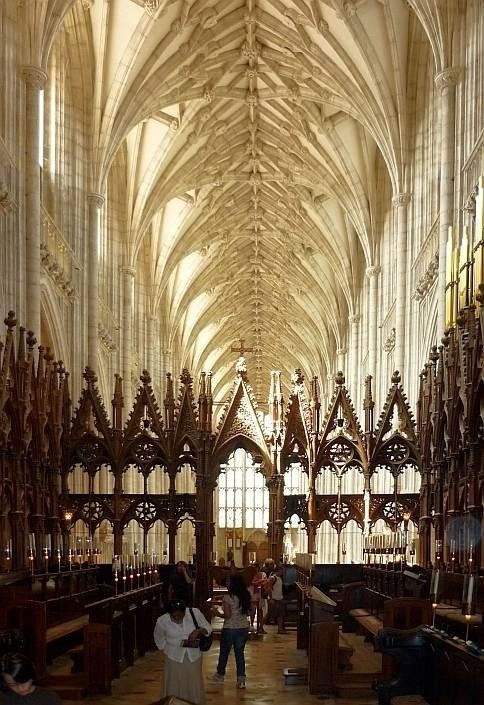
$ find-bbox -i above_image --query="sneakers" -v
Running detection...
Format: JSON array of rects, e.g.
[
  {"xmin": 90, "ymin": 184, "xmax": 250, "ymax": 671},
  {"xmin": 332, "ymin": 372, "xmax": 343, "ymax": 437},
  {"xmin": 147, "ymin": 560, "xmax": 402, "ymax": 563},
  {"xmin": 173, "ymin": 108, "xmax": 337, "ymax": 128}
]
[
  {"xmin": 235, "ymin": 679, "xmax": 247, "ymax": 687},
  {"xmin": 206, "ymin": 674, "xmax": 225, "ymax": 682}
]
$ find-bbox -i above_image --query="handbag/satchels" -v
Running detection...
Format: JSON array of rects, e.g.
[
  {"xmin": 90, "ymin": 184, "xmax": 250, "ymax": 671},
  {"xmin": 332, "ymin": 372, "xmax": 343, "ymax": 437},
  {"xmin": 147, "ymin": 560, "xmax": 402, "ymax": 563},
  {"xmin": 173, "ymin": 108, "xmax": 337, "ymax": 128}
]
[{"xmin": 200, "ymin": 633, "xmax": 213, "ymax": 651}]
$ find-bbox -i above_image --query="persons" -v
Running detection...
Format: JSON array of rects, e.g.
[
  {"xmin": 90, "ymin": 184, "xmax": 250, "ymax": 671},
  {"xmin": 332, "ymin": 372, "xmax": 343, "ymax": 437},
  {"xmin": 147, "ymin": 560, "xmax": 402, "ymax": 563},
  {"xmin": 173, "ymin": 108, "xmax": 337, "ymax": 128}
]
[
  {"xmin": 0, "ymin": 650, "xmax": 54, "ymax": 704},
  {"xmin": 168, "ymin": 559, "xmax": 192, "ymax": 606},
  {"xmin": 250, "ymin": 557, "xmax": 288, "ymax": 635},
  {"xmin": 212, "ymin": 574, "xmax": 251, "ymax": 689},
  {"xmin": 153, "ymin": 599, "xmax": 213, "ymax": 704}
]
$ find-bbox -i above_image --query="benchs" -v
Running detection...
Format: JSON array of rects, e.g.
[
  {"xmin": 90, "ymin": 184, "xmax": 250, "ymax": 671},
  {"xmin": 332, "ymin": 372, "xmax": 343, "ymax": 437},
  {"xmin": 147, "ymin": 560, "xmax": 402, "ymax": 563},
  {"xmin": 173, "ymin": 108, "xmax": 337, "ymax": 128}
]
[
  {"xmin": 294, "ymin": 580, "xmax": 434, "ymax": 695},
  {"xmin": 5, "ymin": 571, "xmax": 165, "ymax": 701}
]
